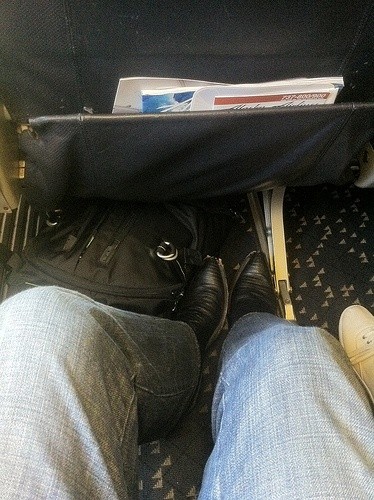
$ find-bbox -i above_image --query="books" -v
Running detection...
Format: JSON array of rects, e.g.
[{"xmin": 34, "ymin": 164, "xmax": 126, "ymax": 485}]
[{"xmin": 113, "ymin": 77, "xmax": 345, "ymax": 112}]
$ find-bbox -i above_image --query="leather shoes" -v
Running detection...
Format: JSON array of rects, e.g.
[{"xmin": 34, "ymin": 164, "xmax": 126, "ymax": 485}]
[
  {"xmin": 169, "ymin": 255, "xmax": 228, "ymax": 421},
  {"xmin": 226, "ymin": 251, "xmax": 282, "ymax": 334}
]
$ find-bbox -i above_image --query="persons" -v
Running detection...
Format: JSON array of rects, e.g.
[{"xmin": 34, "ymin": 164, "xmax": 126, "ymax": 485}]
[
  {"xmin": 339, "ymin": 304, "xmax": 373, "ymax": 405},
  {"xmin": 1, "ymin": 250, "xmax": 373, "ymax": 499}
]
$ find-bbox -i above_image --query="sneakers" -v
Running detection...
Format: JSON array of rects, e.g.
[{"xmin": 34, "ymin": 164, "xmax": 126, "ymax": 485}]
[{"xmin": 339, "ymin": 304, "xmax": 374, "ymax": 402}]
